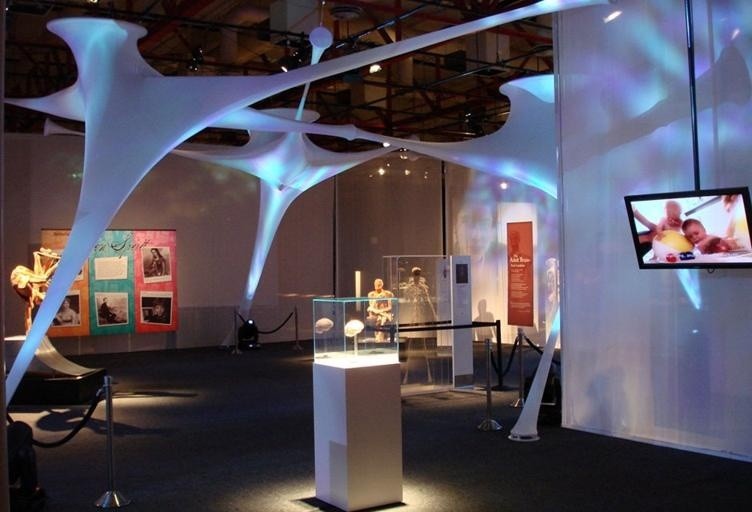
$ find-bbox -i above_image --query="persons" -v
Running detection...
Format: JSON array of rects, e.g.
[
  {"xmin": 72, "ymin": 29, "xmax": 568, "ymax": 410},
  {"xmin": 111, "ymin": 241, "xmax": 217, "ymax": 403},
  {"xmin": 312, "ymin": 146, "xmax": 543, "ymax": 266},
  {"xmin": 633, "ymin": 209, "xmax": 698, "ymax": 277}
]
[
  {"xmin": 405, "ymin": 267, "xmax": 436, "ymax": 324},
  {"xmin": 367, "ymin": 279, "xmax": 394, "ymax": 343},
  {"xmin": 630, "ymin": 204, "xmax": 657, "ymax": 244},
  {"xmin": 55, "ymin": 298, "xmax": 78, "ymax": 326},
  {"xmin": 682, "ymin": 219, "xmax": 722, "ymax": 255},
  {"xmin": 455, "ymin": 196, "xmax": 507, "ymax": 287},
  {"xmin": 144, "ymin": 248, "xmax": 166, "ymax": 277},
  {"xmin": 657, "ymin": 201, "xmax": 683, "ymax": 240},
  {"xmin": 31, "ymin": 296, "xmax": 43, "ymax": 324},
  {"xmin": 101, "ymin": 298, "xmax": 116, "ymax": 323},
  {"xmin": 149, "ymin": 299, "xmax": 170, "ymax": 323}
]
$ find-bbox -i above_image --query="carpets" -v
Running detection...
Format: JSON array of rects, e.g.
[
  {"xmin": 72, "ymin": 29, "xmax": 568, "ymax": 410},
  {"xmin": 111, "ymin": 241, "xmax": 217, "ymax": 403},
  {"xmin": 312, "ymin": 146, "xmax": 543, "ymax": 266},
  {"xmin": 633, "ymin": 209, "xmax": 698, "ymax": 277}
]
[{"xmin": 5, "ymin": 338, "xmax": 750, "ymax": 512}]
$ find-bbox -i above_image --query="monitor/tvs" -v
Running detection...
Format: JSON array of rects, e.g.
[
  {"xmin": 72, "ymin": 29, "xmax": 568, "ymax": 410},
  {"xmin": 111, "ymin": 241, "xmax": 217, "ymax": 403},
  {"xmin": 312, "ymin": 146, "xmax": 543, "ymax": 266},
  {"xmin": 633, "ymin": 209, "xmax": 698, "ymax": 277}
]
[{"xmin": 623, "ymin": 186, "xmax": 752, "ymax": 270}]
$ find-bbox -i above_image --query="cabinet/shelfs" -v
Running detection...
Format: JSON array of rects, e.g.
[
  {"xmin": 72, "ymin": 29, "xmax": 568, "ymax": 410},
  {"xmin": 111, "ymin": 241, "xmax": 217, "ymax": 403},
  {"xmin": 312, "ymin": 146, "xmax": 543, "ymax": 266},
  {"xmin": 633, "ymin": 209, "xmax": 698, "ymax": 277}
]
[
  {"xmin": 311, "ymin": 298, "xmax": 405, "ymax": 510},
  {"xmin": 383, "ymin": 255, "xmax": 471, "ymax": 393}
]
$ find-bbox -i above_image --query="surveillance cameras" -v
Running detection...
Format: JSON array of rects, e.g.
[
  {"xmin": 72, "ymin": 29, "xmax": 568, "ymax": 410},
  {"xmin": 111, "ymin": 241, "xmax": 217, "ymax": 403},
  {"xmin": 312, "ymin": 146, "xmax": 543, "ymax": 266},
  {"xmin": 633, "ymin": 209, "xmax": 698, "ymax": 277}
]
[{"xmin": 187, "ymin": 58, "xmax": 197, "ymax": 72}]
